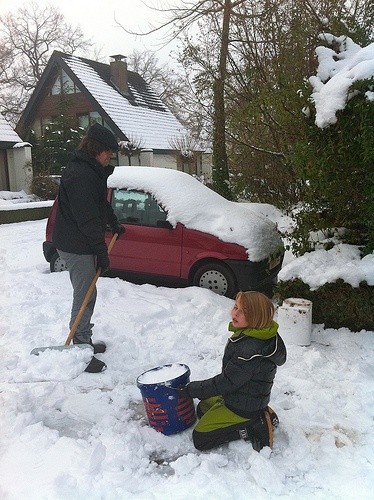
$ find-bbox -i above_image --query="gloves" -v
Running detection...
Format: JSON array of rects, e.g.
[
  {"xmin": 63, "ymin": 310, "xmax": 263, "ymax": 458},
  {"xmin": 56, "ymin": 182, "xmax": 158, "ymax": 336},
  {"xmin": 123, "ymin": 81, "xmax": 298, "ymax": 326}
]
[
  {"xmin": 96, "ymin": 251, "xmax": 109, "ymax": 276},
  {"xmin": 111, "ymin": 219, "xmax": 125, "ymax": 240},
  {"xmin": 177, "ymin": 383, "xmax": 187, "ymax": 397}
]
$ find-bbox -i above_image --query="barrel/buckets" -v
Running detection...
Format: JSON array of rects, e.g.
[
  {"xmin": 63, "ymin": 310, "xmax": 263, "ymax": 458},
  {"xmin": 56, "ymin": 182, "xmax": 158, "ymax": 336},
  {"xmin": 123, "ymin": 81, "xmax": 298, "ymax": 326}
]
[{"xmin": 137, "ymin": 364, "xmax": 196, "ymax": 435}]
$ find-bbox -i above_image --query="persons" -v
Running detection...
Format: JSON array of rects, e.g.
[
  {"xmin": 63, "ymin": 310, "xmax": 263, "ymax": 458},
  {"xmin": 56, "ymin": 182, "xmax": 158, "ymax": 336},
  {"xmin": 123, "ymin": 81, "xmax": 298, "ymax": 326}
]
[
  {"xmin": 188, "ymin": 290, "xmax": 288, "ymax": 455},
  {"xmin": 54, "ymin": 122, "xmax": 125, "ymax": 373}
]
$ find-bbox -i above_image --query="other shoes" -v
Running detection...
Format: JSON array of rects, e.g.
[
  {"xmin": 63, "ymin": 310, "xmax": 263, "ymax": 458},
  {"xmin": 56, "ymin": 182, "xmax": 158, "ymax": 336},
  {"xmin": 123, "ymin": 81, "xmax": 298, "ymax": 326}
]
[
  {"xmin": 85, "ymin": 356, "xmax": 107, "ymax": 373},
  {"xmin": 263, "ymin": 406, "xmax": 279, "ymax": 427},
  {"xmin": 247, "ymin": 411, "xmax": 273, "ymax": 452},
  {"xmin": 73, "ymin": 338, "xmax": 106, "ymax": 354}
]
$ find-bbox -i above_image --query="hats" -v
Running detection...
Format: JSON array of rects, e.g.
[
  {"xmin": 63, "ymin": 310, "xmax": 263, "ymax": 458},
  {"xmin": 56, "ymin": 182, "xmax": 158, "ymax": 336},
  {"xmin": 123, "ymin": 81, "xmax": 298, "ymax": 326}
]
[{"xmin": 88, "ymin": 123, "xmax": 119, "ymax": 150}]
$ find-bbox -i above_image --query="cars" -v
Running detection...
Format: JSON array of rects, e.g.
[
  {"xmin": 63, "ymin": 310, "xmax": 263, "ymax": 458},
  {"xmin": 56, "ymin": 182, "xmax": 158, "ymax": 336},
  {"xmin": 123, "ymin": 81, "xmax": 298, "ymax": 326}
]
[{"xmin": 43, "ymin": 167, "xmax": 285, "ymax": 301}]
